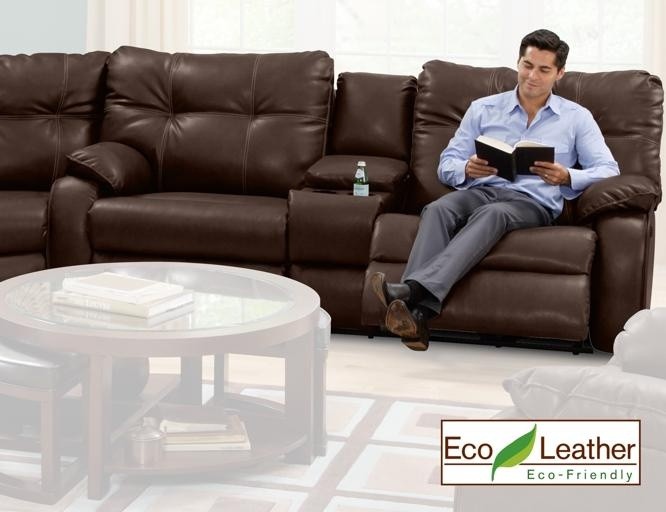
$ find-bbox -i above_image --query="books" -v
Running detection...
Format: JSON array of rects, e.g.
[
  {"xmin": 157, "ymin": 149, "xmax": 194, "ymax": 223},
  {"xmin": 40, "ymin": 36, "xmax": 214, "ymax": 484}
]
[
  {"xmin": 162, "ymin": 413, "xmax": 246, "ymax": 442},
  {"xmin": 53, "ymin": 290, "xmax": 195, "ymax": 320},
  {"xmin": 155, "ymin": 405, "xmax": 230, "ymax": 433},
  {"xmin": 474, "ymin": 135, "xmax": 556, "ymax": 183},
  {"xmin": 164, "ymin": 420, "xmax": 250, "ymax": 452},
  {"xmin": 60, "ymin": 271, "xmax": 183, "ymax": 306}
]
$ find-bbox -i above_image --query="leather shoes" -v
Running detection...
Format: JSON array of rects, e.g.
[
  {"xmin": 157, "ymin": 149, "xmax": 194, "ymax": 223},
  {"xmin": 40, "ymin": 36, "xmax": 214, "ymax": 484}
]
[
  {"xmin": 370, "ymin": 272, "xmax": 412, "ymax": 311},
  {"xmin": 385, "ymin": 299, "xmax": 430, "ymax": 351}
]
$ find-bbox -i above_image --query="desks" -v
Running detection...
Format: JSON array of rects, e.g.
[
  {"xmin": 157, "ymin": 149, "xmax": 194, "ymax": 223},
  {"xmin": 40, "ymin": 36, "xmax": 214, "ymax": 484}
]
[{"xmin": 0, "ymin": 258, "xmax": 331, "ymax": 504}]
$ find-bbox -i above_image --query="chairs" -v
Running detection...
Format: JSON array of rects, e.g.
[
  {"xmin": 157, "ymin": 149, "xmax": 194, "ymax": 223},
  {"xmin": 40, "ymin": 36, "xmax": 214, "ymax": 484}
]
[{"xmin": 452, "ymin": 309, "xmax": 663, "ymax": 512}]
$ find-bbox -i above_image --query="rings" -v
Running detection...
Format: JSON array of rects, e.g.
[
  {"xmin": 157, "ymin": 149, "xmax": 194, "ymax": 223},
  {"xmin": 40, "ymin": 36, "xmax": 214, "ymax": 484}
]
[{"xmin": 545, "ymin": 174, "xmax": 550, "ymax": 179}]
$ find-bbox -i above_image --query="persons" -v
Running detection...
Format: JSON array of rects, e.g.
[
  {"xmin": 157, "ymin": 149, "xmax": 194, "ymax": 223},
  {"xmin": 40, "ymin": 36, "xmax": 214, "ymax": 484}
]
[{"xmin": 370, "ymin": 29, "xmax": 621, "ymax": 350}]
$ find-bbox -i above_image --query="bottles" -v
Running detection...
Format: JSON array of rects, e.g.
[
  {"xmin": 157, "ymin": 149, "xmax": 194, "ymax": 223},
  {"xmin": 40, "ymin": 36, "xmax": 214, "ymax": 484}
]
[{"xmin": 353, "ymin": 160, "xmax": 370, "ymax": 197}]
